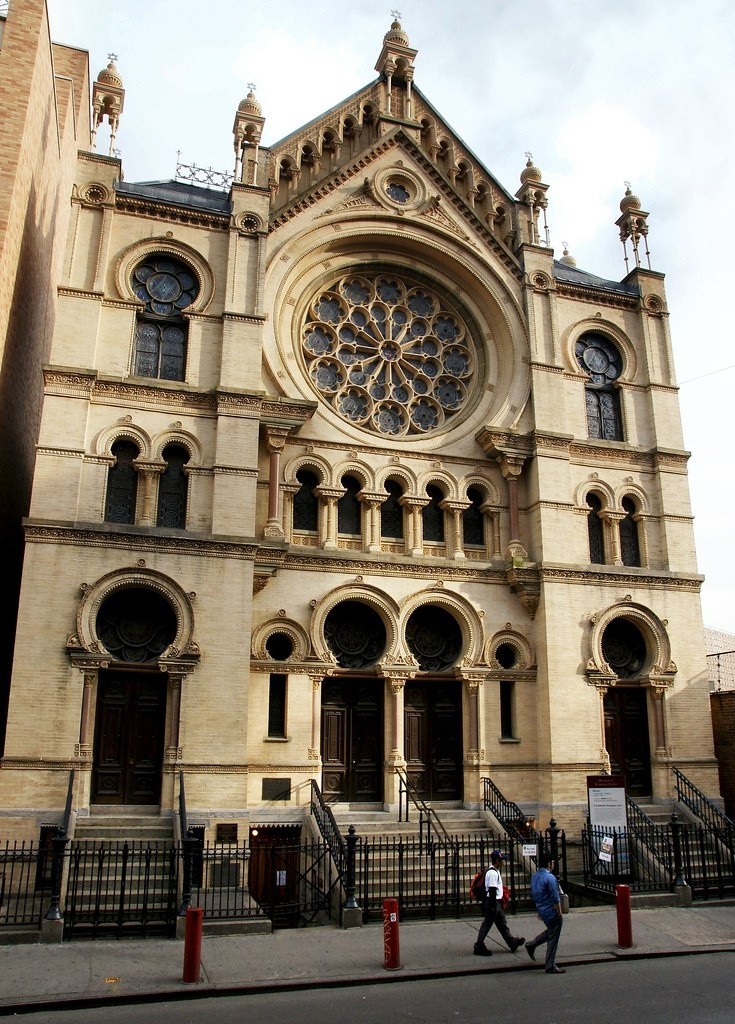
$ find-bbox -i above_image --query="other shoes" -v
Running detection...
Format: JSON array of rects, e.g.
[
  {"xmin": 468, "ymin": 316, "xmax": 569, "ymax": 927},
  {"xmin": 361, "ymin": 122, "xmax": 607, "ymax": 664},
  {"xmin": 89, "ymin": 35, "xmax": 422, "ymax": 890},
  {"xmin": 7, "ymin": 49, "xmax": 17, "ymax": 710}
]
[
  {"xmin": 546, "ymin": 964, "xmax": 565, "ymax": 973},
  {"xmin": 474, "ymin": 942, "xmax": 491, "ymax": 956},
  {"xmin": 511, "ymin": 937, "xmax": 525, "ymax": 953},
  {"xmin": 525, "ymin": 943, "xmax": 536, "ymax": 961}
]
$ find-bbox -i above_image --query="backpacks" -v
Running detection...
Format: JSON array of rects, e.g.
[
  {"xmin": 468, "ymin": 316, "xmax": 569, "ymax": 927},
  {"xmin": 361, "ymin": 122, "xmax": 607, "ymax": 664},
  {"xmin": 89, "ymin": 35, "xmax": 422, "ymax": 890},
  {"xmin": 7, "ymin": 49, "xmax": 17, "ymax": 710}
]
[{"xmin": 470, "ymin": 867, "xmax": 499, "ymax": 900}]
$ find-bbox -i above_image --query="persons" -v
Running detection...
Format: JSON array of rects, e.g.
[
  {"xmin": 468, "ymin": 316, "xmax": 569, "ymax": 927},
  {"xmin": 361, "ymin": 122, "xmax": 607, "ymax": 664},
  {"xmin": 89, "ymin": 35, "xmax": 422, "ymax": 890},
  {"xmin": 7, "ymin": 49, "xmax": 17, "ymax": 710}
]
[
  {"xmin": 525, "ymin": 853, "xmax": 567, "ymax": 973},
  {"xmin": 473, "ymin": 850, "xmax": 526, "ymax": 956}
]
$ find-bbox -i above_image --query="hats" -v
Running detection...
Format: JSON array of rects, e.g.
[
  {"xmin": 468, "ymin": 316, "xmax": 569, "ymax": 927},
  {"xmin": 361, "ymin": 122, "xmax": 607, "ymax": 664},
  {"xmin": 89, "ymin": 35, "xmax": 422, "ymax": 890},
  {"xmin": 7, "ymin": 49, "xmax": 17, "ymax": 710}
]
[{"xmin": 491, "ymin": 850, "xmax": 507, "ymax": 859}]
